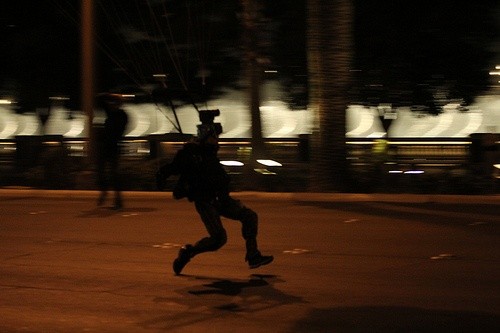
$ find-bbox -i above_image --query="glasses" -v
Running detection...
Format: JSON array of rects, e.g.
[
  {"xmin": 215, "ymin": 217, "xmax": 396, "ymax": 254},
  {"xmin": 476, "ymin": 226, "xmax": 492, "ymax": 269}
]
[{"xmin": 209, "ymin": 134, "xmax": 220, "ymax": 141}]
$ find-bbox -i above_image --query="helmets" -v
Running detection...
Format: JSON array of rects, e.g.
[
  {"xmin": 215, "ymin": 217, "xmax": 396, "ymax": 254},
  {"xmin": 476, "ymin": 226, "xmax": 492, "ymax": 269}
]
[{"xmin": 196, "ymin": 123, "xmax": 222, "ymax": 139}]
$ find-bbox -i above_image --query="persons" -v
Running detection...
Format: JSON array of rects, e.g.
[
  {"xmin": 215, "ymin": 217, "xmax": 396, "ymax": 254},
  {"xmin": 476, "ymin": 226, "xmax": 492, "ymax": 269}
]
[
  {"xmin": 172, "ymin": 109, "xmax": 274, "ymax": 276},
  {"xmin": 94, "ymin": 92, "xmax": 129, "ymax": 209}
]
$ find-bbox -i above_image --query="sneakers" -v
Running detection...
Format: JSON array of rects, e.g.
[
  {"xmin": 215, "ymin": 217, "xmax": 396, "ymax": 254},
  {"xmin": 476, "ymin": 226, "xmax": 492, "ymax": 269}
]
[
  {"xmin": 249, "ymin": 256, "xmax": 274, "ymax": 268},
  {"xmin": 173, "ymin": 246, "xmax": 191, "ymax": 275}
]
